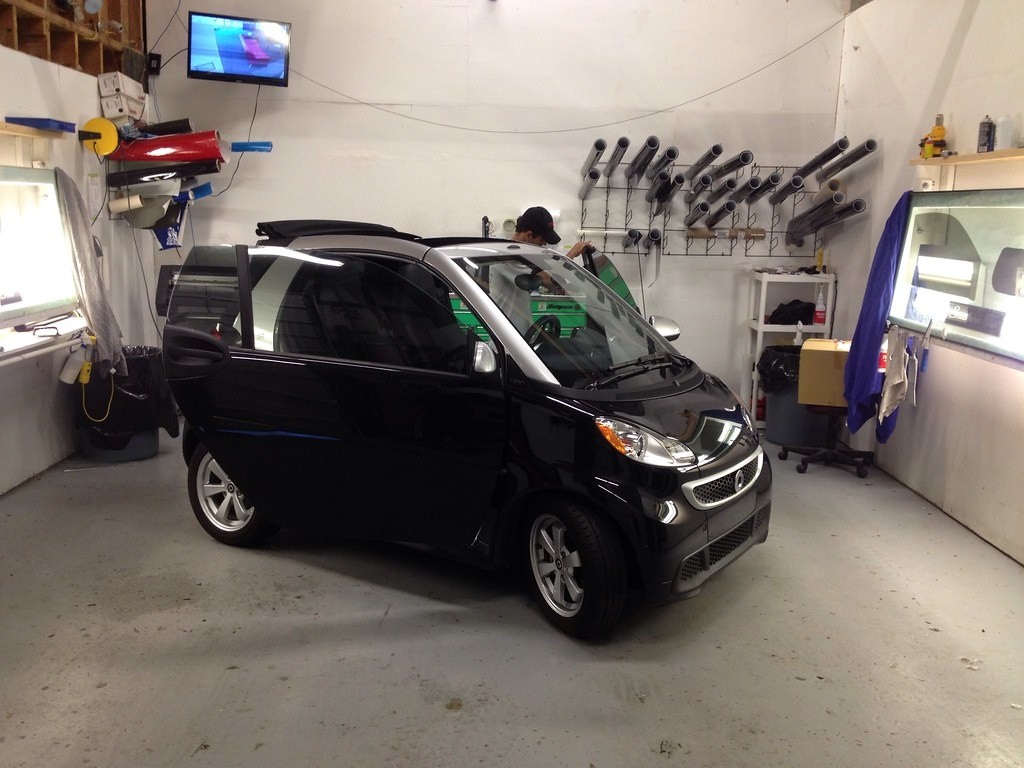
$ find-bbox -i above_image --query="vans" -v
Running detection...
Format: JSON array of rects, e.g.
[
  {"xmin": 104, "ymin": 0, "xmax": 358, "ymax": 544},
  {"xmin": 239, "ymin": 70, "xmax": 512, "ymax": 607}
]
[{"xmin": 160, "ymin": 220, "xmax": 777, "ymax": 647}]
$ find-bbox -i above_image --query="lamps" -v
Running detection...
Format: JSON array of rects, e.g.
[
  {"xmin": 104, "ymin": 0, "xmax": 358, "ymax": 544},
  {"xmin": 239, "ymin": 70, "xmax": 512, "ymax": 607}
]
[{"xmin": 98, "ymin": 19, "xmax": 124, "ymax": 33}]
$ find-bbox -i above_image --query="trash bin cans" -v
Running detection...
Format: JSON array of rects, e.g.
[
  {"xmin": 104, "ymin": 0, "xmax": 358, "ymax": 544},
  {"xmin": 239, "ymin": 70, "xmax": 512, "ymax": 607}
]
[
  {"xmin": 764, "ymin": 345, "xmax": 830, "ymax": 448},
  {"xmin": 76, "ymin": 345, "xmax": 163, "ymax": 464}
]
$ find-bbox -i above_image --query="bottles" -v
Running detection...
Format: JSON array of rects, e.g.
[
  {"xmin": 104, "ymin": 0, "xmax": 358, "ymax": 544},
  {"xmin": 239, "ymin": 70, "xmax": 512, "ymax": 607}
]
[
  {"xmin": 817, "ymin": 248, "xmax": 831, "ymax": 272},
  {"xmin": 977, "ymin": 115, "xmax": 995, "ymax": 152},
  {"xmin": 924, "ymin": 138, "xmax": 934, "ymax": 159}
]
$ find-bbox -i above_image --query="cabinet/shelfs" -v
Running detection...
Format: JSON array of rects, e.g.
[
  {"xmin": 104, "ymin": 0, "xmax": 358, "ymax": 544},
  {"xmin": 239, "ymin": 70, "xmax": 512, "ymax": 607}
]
[
  {"xmin": 0, "ymin": 0, "xmax": 143, "ymax": 82},
  {"xmin": 745, "ymin": 268, "xmax": 836, "ymax": 430}
]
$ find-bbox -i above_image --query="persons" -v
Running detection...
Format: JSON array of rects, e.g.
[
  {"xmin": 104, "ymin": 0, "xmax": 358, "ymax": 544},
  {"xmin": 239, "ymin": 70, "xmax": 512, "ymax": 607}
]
[{"xmin": 488, "ymin": 206, "xmax": 593, "ymax": 342}]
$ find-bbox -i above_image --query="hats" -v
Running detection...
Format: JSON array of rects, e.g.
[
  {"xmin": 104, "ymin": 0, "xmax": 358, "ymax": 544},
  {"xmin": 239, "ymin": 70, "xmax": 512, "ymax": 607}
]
[{"xmin": 516, "ymin": 206, "xmax": 561, "ymax": 244}]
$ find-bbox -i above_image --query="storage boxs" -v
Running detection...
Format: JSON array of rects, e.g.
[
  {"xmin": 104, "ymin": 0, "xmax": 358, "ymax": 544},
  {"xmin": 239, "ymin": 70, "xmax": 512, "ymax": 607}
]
[
  {"xmin": 98, "ymin": 71, "xmax": 148, "ymax": 123},
  {"xmin": 798, "ymin": 337, "xmax": 853, "ymax": 408}
]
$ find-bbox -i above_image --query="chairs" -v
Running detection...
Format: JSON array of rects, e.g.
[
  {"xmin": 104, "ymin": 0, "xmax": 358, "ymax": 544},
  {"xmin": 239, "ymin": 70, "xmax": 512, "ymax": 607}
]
[{"xmin": 302, "ymin": 277, "xmax": 449, "ymax": 372}]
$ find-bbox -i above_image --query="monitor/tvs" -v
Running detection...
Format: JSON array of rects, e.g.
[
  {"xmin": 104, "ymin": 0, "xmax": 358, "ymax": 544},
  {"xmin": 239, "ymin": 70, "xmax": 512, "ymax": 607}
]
[{"xmin": 186, "ymin": 10, "xmax": 292, "ymax": 87}]
